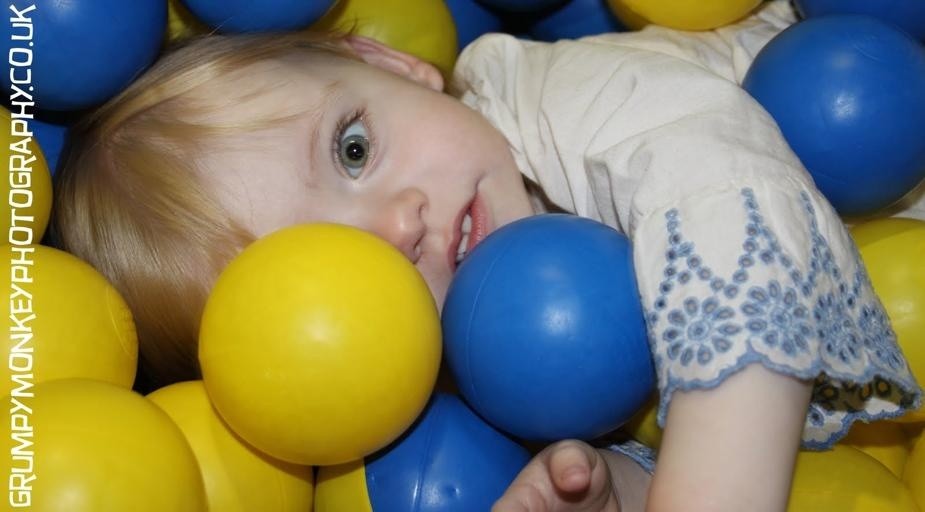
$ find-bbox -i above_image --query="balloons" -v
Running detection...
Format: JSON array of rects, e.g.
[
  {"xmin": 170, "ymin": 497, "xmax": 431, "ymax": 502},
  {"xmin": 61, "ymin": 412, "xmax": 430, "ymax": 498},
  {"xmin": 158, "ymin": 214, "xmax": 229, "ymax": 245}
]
[
  {"xmin": 0, "ymin": 215, "xmax": 655, "ymax": 511},
  {"xmin": 1, "ymin": 1, "xmax": 606, "ymax": 106},
  {"xmin": 618, "ymin": 0, "xmax": 925, "ymax": 511}
]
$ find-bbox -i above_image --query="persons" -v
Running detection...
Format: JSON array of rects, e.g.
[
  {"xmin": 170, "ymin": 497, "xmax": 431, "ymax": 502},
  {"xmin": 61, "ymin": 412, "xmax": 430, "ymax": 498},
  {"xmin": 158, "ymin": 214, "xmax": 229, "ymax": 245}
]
[
  {"xmin": 52, "ymin": 0, "xmax": 925, "ymax": 512},
  {"xmin": 1, "ymin": 214, "xmax": 654, "ymax": 509}
]
